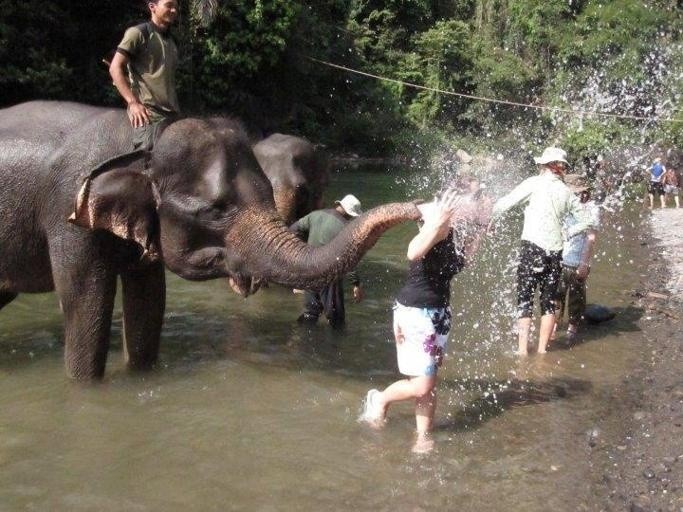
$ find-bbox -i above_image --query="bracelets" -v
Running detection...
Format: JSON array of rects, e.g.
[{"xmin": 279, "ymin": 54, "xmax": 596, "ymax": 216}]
[
  {"xmin": 127, "ymin": 101, "xmax": 141, "ymax": 110},
  {"xmin": 580, "ymin": 262, "xmax": 591, "ymax": 268}
]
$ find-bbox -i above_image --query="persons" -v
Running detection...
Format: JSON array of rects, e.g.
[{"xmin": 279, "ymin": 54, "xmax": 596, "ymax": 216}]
[
  {"xmin": 361, "ymin": 174, "xmax": 495, "ymax": 457},
  {"xmin": 645, "ymin": 157, "xmax": 669, "ymax": 209},
  {"xmin": 662, "ymin": 168, "xmax": 680, "ymax": 209},
  {"xmin": 287, "ymin": 192, "xmax": 366, "ymax": 329},
  {"xmin": 491, "ymin": 138, "xmax": 578, "ymax": 359},
  {"xmin": 545, "ymin": 173, "xmax": 601, "ymax": 347},
  {"xmin": 108, "ymin": 0, "xmax": 184, "ymax": 131}
]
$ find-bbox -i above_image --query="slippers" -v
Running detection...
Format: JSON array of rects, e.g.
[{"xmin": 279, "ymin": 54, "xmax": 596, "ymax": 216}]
[{"xmin": 365, "ymin": 386, "xmax": 389, "ymax": 428}]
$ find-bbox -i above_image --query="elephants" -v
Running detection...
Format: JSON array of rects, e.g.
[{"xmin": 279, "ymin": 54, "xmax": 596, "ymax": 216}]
[{"xmin": 0, "ymin": 98, "xmax": 423, "ymax": 380}]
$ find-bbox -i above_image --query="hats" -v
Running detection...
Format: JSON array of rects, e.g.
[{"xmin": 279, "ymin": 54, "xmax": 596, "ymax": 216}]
[
  {"xmin": 334, "ymin": 193, "xmax": 362, "ymax": 218},
  {"xmin": 563, "ymin": 174, "xmax": 595, "ymax": 194},
  {"xmin": 532, "ymin": 145, "xmax": 572, "ymax": 168}
]
